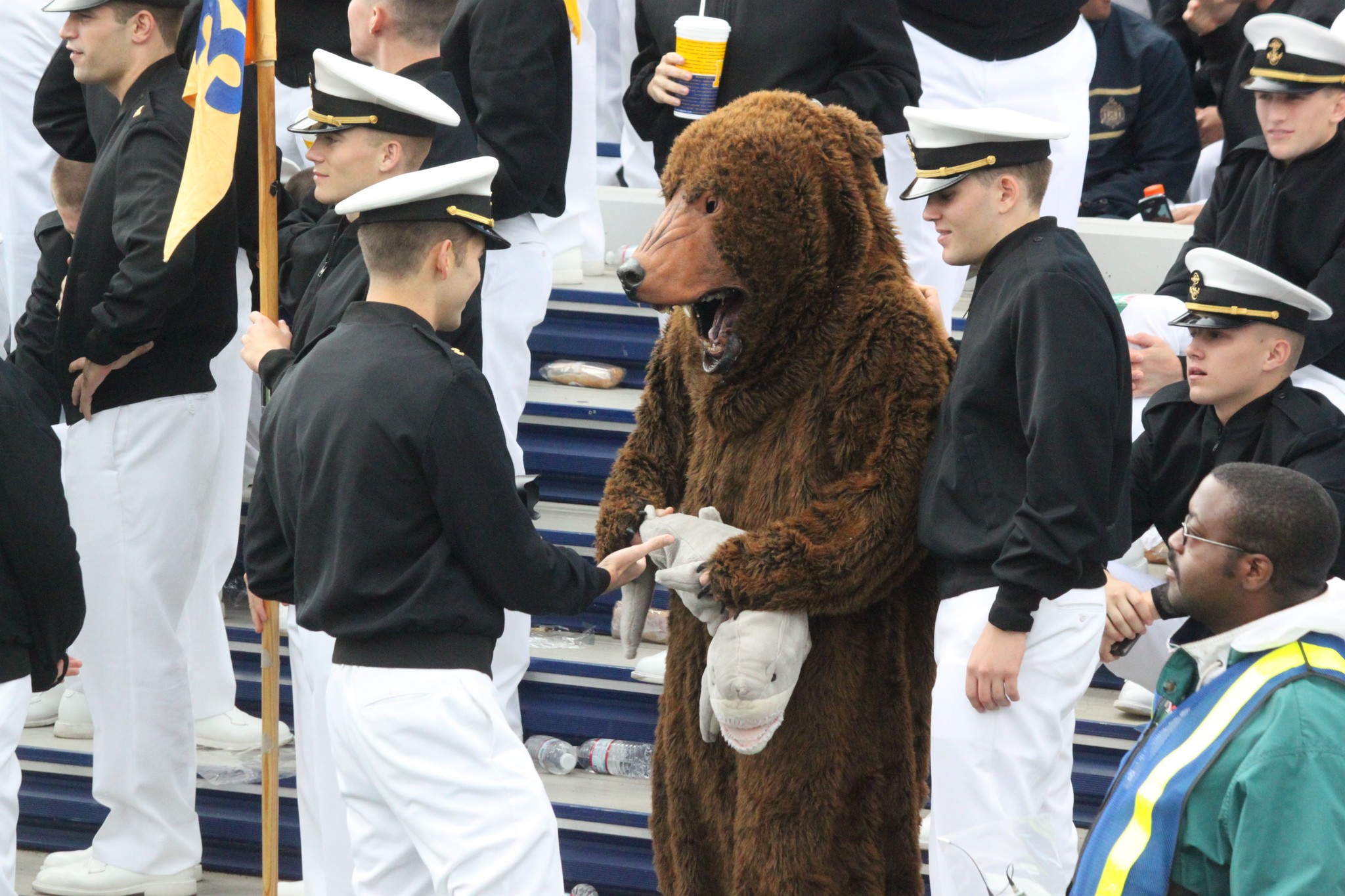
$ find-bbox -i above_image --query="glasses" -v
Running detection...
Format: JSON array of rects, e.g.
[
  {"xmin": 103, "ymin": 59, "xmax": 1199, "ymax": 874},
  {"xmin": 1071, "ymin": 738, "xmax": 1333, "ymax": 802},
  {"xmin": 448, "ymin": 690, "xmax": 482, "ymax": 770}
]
[{"xmin": 1182, "ymin": 513, "xmax": 1258, "ymax": 554}]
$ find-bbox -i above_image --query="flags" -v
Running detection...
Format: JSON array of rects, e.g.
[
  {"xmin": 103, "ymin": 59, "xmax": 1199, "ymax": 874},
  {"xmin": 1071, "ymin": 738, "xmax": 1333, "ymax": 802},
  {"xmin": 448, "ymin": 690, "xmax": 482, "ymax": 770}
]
[{"xmin": 163, "ymin": 0, "xmax": 277, "ymax": 263}]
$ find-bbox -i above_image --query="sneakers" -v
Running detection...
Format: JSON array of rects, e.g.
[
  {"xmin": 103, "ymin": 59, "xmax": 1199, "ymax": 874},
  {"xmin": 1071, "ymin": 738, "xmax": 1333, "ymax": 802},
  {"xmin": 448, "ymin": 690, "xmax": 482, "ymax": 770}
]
[
  {"xmin": 631, "ymin": 649, "xmax": 668, "ymax": 684},
  {"xmin": 918, "ymin": 813, "xmax": 930, "ymax": 849},
  {"xmin": 552, "ymin": 248, "xmax": 605, "ymax": 286}
]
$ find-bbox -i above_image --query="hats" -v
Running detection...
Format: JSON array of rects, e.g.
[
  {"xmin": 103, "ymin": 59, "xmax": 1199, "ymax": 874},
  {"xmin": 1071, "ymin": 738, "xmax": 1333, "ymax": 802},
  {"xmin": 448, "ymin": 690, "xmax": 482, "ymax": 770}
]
[
  {"xmin": 286, "ymin": 48, "xmax": 461, "ymax": 138},
  {"xmin": 1167, "ymin": 248, "xmax": 1334, "ymax": 334},
  {"xmin": 41, "ymin": 1, "xmax": 110, "ymax": 12},
  {"xmin": 899, "ymin": 106, "xmax": 1071, "ymax": 201},
  {"xmin": 1240, "ymin": 13, "xmax": 1345, "ymax": 95},
  {"xmin": 334, "ymin": 156, "xmax": 511, "ymax": 250}
]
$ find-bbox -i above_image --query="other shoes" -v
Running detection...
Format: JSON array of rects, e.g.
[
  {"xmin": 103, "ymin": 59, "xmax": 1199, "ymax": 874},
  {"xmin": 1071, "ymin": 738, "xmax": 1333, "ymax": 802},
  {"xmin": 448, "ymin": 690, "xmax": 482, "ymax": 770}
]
[
  {"xmin": 54, "ymin": 688, "xmax": 94, "ymax": 739},
  {"xmin": 23, "ymin": 682, "xmax": 67, "ymax": 728},
  {"xmin": 1113, "ymin": 680, "xmax": 1154, "ymax": 716},
  {"xmin": 33, "ymin": 845, "xmax": 204, "ymax": 896},
  {"xmin": 195, "ymin": 706, "xmax": 294, "ymax": 750}
]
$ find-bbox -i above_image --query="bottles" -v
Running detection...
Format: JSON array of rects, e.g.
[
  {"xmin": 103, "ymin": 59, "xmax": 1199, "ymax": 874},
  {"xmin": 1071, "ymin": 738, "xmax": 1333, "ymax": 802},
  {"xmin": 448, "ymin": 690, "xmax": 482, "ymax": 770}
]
[
  {"xmin": 572, "ymin": 738, "xmax": 654, "ymax": 779},
  {"xmin": 604, "ymin": 244, "xmax": 636, "ymax": 264},
  {"xmin": 527, "ymin": 735, "xmax": 579, "ymax": 776},
  {"xmin": 1144, "ymin": 184, "xmax": 1174, "ymax": 209}
]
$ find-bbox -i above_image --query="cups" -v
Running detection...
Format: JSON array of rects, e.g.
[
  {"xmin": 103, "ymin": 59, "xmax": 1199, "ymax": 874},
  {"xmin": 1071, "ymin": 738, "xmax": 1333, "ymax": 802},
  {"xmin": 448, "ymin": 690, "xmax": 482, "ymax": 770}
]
[{"xmin": 672, "ymin": 14, "xmax": 731, "ymax": 119}]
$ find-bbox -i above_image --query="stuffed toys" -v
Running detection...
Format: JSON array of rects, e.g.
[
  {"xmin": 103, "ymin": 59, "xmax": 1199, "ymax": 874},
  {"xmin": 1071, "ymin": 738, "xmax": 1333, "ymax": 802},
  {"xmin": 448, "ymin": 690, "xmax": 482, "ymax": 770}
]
[
  {"xmin": 597, "ymin": 92, "xmax": 957, "ymax": 896},
  {"xmin": 620, "ymin": 505, "xmax": 812, "ymax": 755}
]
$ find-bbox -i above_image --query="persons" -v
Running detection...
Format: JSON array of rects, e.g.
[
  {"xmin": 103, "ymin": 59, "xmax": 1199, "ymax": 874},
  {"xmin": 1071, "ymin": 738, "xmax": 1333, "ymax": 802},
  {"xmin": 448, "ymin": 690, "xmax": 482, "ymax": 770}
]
[
  {"xmin": 1067, "ymin": 463, "xmax": 1345, "ymax": 896},
  {"xmin": 245, "ymin": 156, "xmax": 676, "ymax": 896},
  {"xmin": 1081, "ymin": 0, "xmax": 1345, "ymax": 716},
  {"xmin": 897, "ymin": 105, "xmax": 1132, "ymax": 896},
  {"xmin": 0, "ymin": 0, "xmax": 240, "ymax": 896},
  {"xmin": 623, "ymin": 0, "xmax": 1097, "ymax": 444},
  {"xmin": 242, "ymin": 0, "xmax": 592, "ymax": 748}
]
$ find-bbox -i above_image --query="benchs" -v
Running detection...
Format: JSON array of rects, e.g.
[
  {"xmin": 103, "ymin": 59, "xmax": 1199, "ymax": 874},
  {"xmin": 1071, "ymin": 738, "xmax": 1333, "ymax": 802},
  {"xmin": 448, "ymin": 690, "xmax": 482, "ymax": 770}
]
[{"xmin": 11, "ymin": 144, "xmax": 1152, "ymax": 896}]
